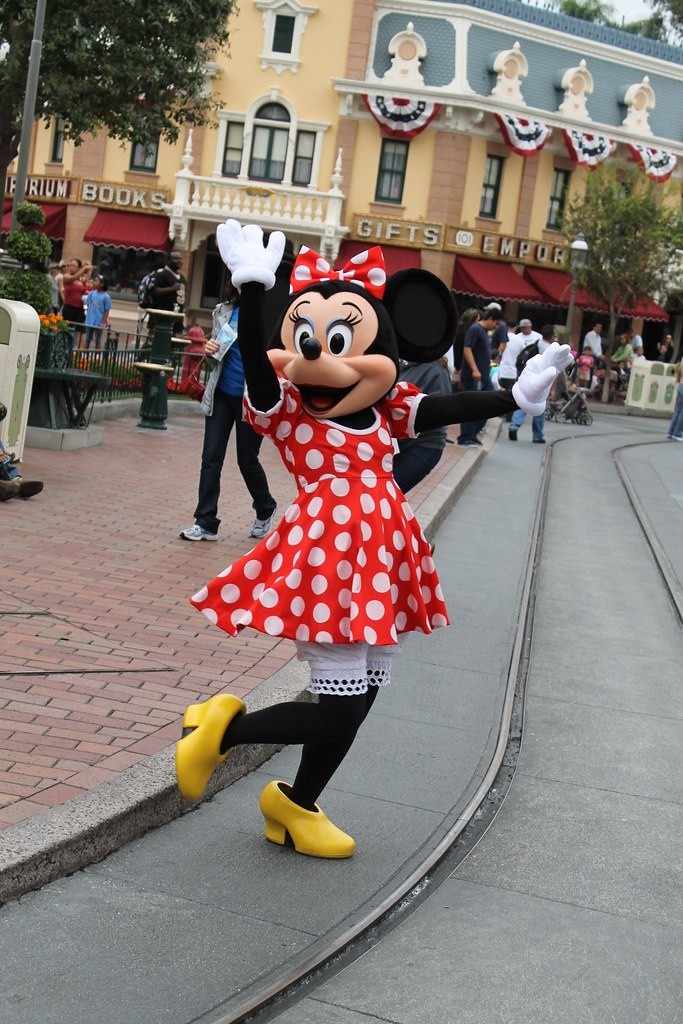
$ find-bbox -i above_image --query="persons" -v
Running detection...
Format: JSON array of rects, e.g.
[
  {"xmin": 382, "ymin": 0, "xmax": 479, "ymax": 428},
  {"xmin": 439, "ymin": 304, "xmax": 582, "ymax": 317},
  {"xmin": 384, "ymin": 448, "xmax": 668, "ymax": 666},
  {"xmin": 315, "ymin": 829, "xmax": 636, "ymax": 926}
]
[
  {"xmin": 667, "ymin": 356, "xmax": 683, "ymax": 443},
  {"xmin": 455, "ymin": 302, "xmax": 556, "ymax": 446},
  {"xmin": 609, "ymin": 327, "xmax": 644, "ymax": 405},
  {"xmin": 83, "ymin": 275, "xmax": 111, "ymax": 362},
  {"xmin": 657, "ymin": 334, "xmax": 675, "ymax": 361},
  {"xmin": 147, "ymin": 251, "xmax": 187, "ymax": 360},
  {"xmin": 46, "ymin": 256, "xmax": 97, "ymax": 347},
  {"xmin": 576, "ymin": 321, "xmax": 607, "ymax": 402},
  {"xmin": 178, "ymin": 278, "xmax": 279, "ymax": 539}
]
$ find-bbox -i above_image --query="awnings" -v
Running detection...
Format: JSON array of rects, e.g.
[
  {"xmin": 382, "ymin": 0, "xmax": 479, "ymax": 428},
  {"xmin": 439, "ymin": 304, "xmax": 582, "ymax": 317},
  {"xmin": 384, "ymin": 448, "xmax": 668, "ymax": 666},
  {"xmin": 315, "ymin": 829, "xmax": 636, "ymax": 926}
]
[
  {"xmin": 82, "ymin": 207, "xmax": 173, "ymax": 257},
  {"xmin": 450, "ymin": 252, "xmax": 541, "ymax": 307},
  {"xmin": 522, "ymin": 264, "xmax": 669, "ymax": 323},
  {"xmin": 332, "ymin": 239, "xmax": 422, "ymax": 281},
  {"xmin": 0, "ymin": 200, "xmax": 68, "ymax": 241}
]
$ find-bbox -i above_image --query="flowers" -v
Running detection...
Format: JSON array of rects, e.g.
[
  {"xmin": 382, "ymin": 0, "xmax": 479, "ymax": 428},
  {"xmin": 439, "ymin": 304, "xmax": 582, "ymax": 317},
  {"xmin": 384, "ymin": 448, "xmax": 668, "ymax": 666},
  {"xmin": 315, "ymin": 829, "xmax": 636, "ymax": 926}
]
[{"xmin": 40, "ymin": 312, "xmax": 73, "ymax": 334}]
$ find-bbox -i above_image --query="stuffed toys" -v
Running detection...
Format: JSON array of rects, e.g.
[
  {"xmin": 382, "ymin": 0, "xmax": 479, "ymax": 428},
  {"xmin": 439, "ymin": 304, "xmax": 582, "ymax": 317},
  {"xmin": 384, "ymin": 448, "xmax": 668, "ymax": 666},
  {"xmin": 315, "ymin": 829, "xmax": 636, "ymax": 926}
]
[{"xmin": 174, "ymin": 217, "xmax": 573, "ymax": 860}]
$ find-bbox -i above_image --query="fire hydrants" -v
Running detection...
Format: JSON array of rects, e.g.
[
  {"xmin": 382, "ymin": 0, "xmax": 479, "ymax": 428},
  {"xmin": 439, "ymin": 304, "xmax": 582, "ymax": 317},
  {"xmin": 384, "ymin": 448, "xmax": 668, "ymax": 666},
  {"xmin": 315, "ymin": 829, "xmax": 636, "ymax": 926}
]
[{"xmin": 180, "ymin": 324, "xmax": 209, "ymax": 386}]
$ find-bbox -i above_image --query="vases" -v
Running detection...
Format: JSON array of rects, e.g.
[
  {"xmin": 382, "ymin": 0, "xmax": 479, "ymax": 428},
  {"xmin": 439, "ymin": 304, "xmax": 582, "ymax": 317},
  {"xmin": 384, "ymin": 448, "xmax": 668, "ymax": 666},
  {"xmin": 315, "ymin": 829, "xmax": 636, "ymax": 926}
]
[{"xmin": 36, "ymin": 330, "xmax": 69, "ymax": 368}]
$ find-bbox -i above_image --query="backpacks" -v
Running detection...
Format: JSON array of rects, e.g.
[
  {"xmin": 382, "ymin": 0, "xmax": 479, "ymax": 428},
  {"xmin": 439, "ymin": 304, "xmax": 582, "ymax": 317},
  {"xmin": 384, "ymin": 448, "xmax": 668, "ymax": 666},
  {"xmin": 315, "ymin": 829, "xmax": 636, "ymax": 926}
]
[{"xmin": 138, "ymin": 269, "xmax": 164, "ymax": 307}]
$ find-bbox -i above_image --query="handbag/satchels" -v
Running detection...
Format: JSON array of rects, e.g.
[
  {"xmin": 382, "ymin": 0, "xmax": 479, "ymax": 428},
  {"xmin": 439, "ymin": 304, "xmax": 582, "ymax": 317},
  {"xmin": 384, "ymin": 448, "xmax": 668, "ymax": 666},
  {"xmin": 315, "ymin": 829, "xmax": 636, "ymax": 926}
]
[{"xmin": 178, "ymin": 375, "xmax": 206, "ymax": 402}]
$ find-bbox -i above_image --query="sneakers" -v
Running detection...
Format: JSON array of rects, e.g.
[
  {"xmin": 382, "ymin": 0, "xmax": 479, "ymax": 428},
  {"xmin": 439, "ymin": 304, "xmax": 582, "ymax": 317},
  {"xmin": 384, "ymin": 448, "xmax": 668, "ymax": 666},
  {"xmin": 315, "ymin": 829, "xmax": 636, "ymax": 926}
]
[
  {"xmin": 179, "ymin": 524, "xmax": 219, "ymax": 541},
  {"xmin": 250, "ymin": 507, "xmax": 278, "ymax": 538}
]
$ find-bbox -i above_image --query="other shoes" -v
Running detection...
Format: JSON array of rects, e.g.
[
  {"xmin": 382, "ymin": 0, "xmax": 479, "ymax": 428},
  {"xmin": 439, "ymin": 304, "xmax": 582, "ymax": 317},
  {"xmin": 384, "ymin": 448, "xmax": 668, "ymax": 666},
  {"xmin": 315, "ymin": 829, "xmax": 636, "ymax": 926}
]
[
  {"xmin": 457, "ymin": 437, "xmax": 482, "ymax": 447},
  {"xmin": 10, "ymin": 476, "xmax": 44, "ymax": 498},
  {"xmin": 508, "ymin": 427, "xmax": 517, "ymax": 440},
  {"xmin": 667, "ymin": 434, "xmax": 683, "ymax": 442},
  {"xmin": 533, "ymin": 438, "xmax": 545, "ymax": 443},
  {"xmin": 0, "ymin": 479, "xmax": 21, "ymax": 502}
]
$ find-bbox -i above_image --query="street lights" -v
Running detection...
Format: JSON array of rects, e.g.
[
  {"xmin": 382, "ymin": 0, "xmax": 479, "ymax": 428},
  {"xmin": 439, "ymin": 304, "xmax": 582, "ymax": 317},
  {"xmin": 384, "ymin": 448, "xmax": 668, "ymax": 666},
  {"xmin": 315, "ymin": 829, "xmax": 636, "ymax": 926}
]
[{"xmin": 563, "ymin": 232, "xmax": 589, "ymax": 347}]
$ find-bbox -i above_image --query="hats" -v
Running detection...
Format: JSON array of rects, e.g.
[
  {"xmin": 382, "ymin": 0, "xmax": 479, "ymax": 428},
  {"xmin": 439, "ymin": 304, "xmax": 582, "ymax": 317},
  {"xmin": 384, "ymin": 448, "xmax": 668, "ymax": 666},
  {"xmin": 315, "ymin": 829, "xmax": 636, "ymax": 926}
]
[
  {"xmin": 518, "ymin": 319, "xmax": 532, "ymax": 328},
  {"xmin": 483, "ymin": 303, "xmax": 502, "ymax": 311}
]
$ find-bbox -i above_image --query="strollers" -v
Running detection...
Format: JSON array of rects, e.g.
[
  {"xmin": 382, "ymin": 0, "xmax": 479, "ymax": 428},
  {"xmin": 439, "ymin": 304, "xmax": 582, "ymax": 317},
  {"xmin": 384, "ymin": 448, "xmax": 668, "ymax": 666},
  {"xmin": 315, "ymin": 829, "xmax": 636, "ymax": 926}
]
[{"xmin": 544, "ymin": 369, "xmax": 593, "ymax": 427}]
